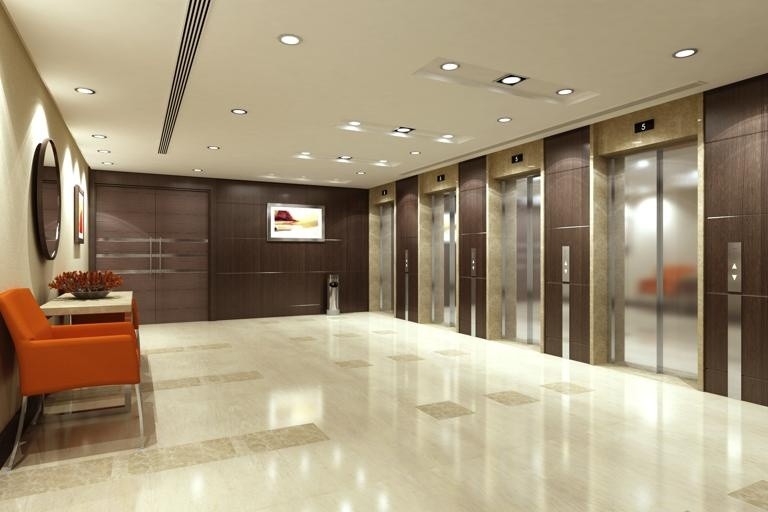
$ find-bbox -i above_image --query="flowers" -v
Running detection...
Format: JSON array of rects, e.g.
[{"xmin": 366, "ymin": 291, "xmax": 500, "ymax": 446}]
[{"xmin": 47, "ymin": 269, "xmax": 124, "ymax": 292}]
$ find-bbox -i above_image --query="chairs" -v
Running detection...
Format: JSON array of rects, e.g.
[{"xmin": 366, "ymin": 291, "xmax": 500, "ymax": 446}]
[{"xmin": 0, "ymin": 287, "xmax": 146, "ymax": 470}]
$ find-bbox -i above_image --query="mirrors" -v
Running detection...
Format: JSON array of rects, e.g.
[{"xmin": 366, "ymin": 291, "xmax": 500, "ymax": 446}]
[{"xmin": 30, "ymin": 137, "xmax": 63, "ymax": 261}]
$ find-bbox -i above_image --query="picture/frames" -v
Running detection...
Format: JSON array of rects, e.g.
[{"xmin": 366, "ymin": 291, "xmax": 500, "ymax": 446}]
[{"xmin": 74, "ymin": 185, "xmax": 87, "ymax": 246}]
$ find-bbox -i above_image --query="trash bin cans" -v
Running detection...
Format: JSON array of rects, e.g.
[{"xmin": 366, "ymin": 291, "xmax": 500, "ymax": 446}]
[{"xmin": 326, "ymin": 273, "xmax": 340, "ymax": 316}]
